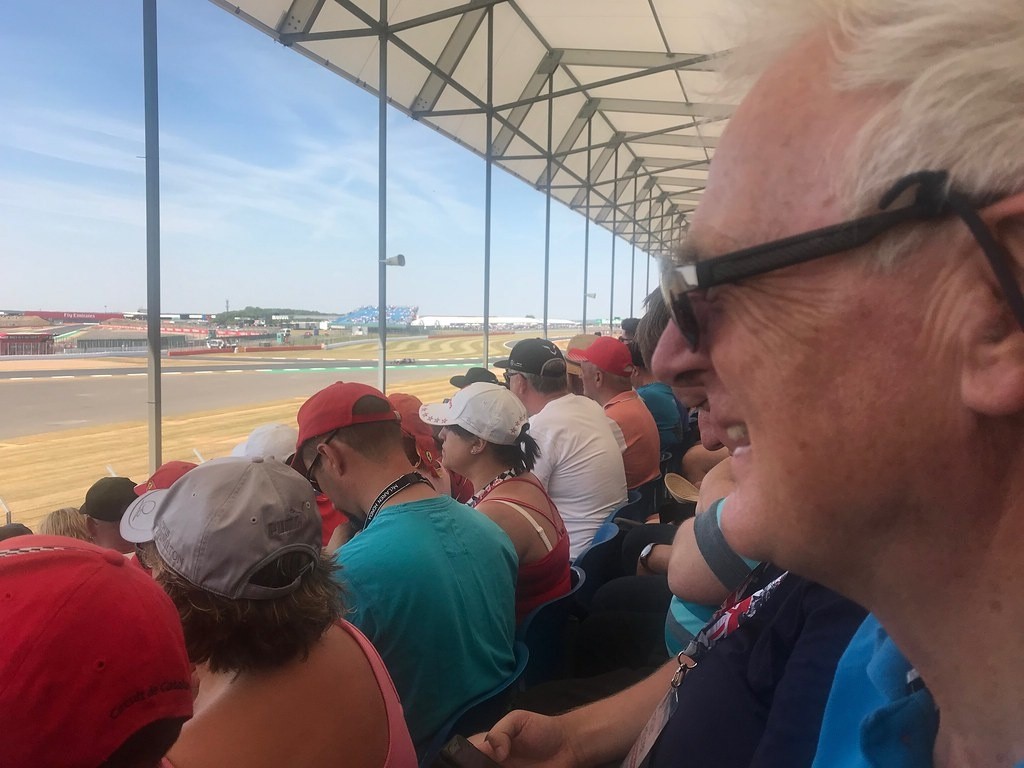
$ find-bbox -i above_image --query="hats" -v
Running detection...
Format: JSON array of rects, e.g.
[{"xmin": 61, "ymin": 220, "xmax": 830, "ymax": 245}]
[
  {"xmin": 290, "ymin": 381, "xmax": 402, "ymax": 475},
  {"xmin": 387, "ymin": 393, "xmax": 441, "ymax": 480},
  {"xmin": 665, "ymin": 472, "xmax": 699, "ymax": 505},
  {"xmin": 0, "ymin": 522, "xmax": 34, "ymax": 544},
  {"xmin": 135, "ymin": 460, "xmax": 198, "ymax": 496},
  {"xmin": 419, "ymin": 382, "xmax": 530, "ymax": 446},
  {"xmin": 567, "ymin": 334, "xmax": 638, "ymax": 378},
  {"xmin": 0, "ymin": 536, "xmax": 199, "ymax": 768},
  {"xmin": 231, "ymin": 423, "xmax": 298, "ymax": 465},
  {"xmin": 449, "ymin": 367, "xmax": 497, "ymax": 389},
  {"xmin": 493, "ymin": 337, "xmax": 565, "ymax": 379},
  {"xmin": 118, "ymin": 457, "xmax": 323, "ymax": 600},
  {"xmin": 79, "ymin": 477, "xmax": 140, "ymax": 522}
]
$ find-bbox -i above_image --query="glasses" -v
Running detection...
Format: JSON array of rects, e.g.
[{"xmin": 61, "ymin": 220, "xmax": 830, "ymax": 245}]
[
  {"xmin": 658, "ymin": 170, "xmax": 1023, "ymax": 356},
  {"xmin": 503, "ymin": 372, "xmax": 517, "ymax": 380},
  {"xmin": 135, "ymin": 543, "xmax": 153, "ymax": 571},
  {"xmin": 302, "ymin": 430, "xmax": 339, "ymax": 493}
]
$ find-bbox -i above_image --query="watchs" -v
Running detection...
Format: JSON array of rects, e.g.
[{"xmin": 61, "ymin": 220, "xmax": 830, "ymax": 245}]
[{"xmin": 641, "ymin": 540, "xmax": 657, "ymax": 573}]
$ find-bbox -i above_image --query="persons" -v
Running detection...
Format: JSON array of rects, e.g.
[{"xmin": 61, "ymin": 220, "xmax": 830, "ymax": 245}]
[
  {"xmin": 295, "ymin": 383, "xmax": 514, "ymax": 768},
  {"xmin": 0, "ymin": 0, "xmax": 1024, "ymax": 768}
]
